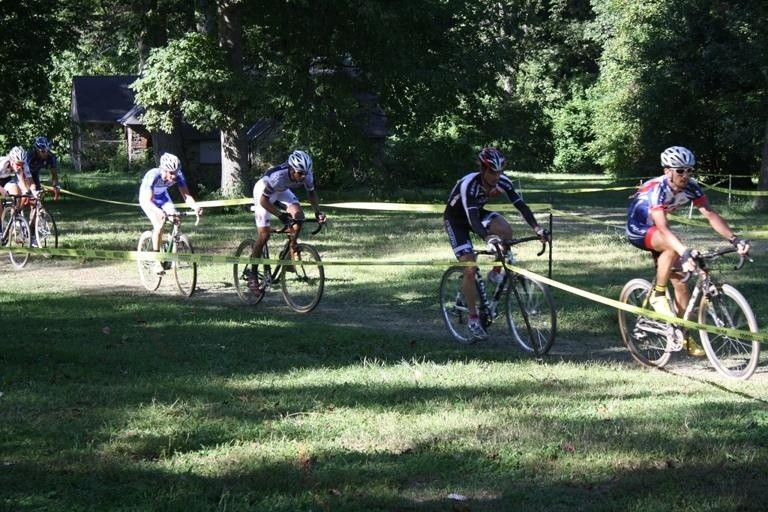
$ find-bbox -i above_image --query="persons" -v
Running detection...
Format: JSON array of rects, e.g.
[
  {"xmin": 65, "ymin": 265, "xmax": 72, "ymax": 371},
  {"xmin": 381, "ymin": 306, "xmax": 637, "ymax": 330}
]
[
  {"xmin": 625, "ymin": 146, "xmax": 750, "ymax": 358},
  {"xmin": 0, "ymin": 146, "xmax": 42, "ymax": 235},
  {"xmin": 248, "ymin": 151, "xmax": 326, "ymax": 297},
  {"xmin": 443, "ymin": 147, "xmax": 550, "ymax": 342},
  {"xmin": 15, "ymin": 137, "xmax": 60, "ymax": 248},
  {"xmin": 138, "ymin": 152, "xmax": 203, "ymax": 276}
]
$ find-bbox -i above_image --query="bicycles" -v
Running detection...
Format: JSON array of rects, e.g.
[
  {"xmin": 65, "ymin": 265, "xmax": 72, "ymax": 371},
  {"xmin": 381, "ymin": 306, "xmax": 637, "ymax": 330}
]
[
  {"xmin": 22, "ymin": 184, "xmax": 60, "ymax": 252},
  {"xmin": 616, "ymin": 241, "xmax": 760, "ymax": 380},
  {"xmin": 136, "ymin": 209, "xmax": 200, "ymax": 297},
  {"xmin": 1, "ymin": 194, "xmax": 38, "ymax": 268},
  {"xmin": 232, "ymin": 217, "xmax": 325, "ymax": 314},
  {"xmin": 438, "ymin": 213, "xmax": 559, "ymax": 364}
]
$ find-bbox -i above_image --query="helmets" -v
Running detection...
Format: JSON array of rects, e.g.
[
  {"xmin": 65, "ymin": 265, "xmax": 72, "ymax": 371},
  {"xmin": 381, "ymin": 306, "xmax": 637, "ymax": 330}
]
[
  {"xmin": 478, "ymin": 147, "xmax": 507, "ymax": 173},
  {"xmin": 34, "ymin": 137, "xmax": 50, "ymax": 150},
  {"xmin": 660, "ymin": 146, "xmax": 696, "ymax": 168},
  {"xmin": 288, "ymin": 149, "xmax": 314, "ymax": 174},
  {"xmin": 8, "ymin": 146, "xmax": 28, "ymax": 164},
  {"xmin": 160, "ymin": 153, "xmax": 182, "ymax": 173}
]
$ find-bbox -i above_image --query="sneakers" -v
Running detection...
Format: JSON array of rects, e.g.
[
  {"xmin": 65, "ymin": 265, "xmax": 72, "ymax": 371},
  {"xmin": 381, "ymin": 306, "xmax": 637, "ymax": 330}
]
[
  {"xmin": 487, "ymin": 269, "xmax": 510, "ymax": 291},
  {"xmin": 649, "ymin": 291, "xmax": 678, "ymax": 319},
  {"xmin": 247, "ymin": 274, "xmax": 261, "ymax": 296},
  {"xmin": 467, "ymin": 320, "xmax": 489, "ymax": 341},
  {"xmin": 682, "ymin": 335, "xmax": 706, "ymax": 357},
  {"xmin": 150, "ymin": 260, "xmax": 166, "ymax": 275}
]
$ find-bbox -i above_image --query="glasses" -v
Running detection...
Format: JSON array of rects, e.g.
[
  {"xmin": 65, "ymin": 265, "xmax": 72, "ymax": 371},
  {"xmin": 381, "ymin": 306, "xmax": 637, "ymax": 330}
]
[
  {"xmin": 670, "ymin": 168, "xmax": 693, "ymax": 175},
  {"xmin": 39, "ymin": 149, "xmax": 49, "ymax": 153}
]
